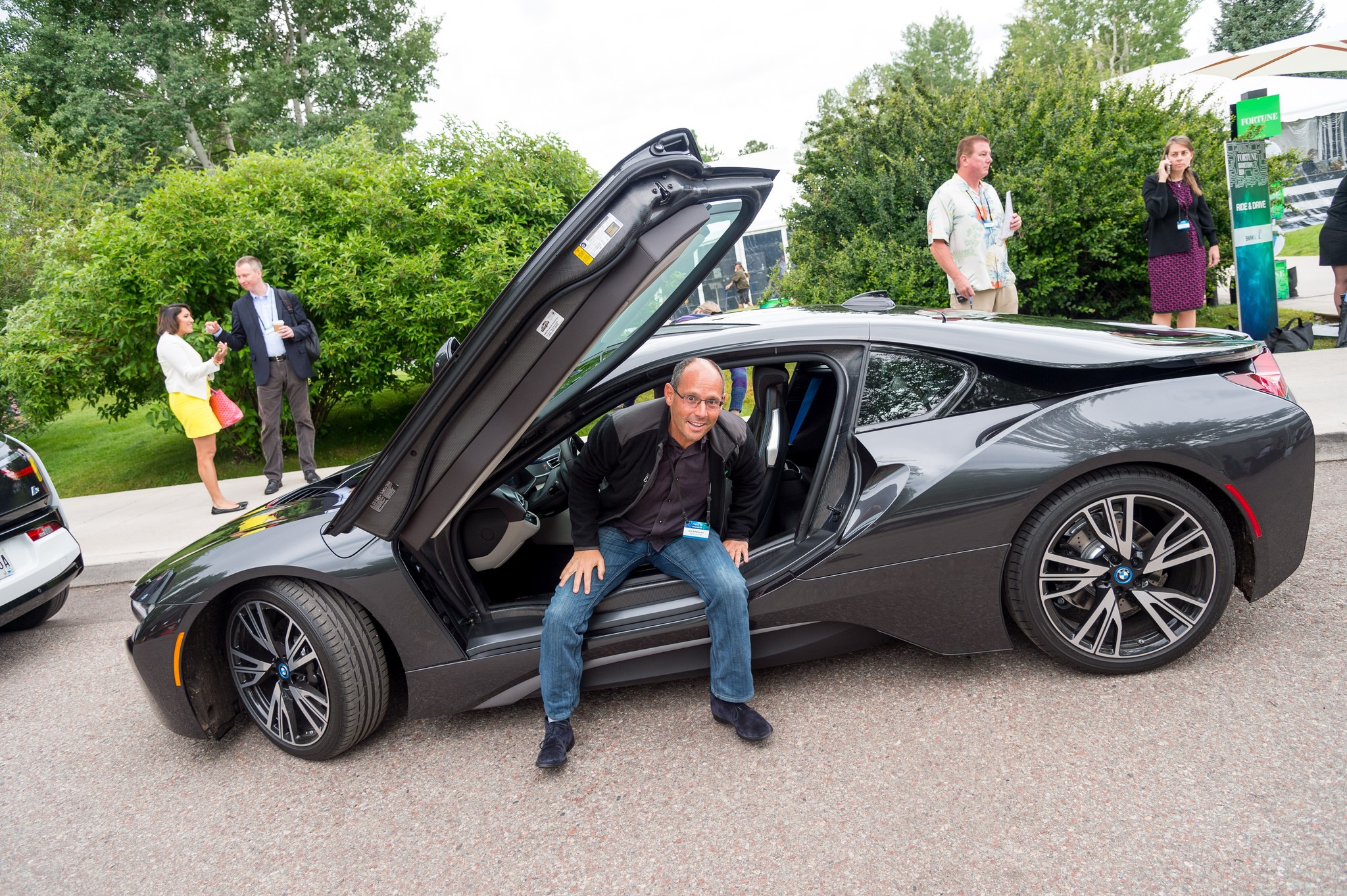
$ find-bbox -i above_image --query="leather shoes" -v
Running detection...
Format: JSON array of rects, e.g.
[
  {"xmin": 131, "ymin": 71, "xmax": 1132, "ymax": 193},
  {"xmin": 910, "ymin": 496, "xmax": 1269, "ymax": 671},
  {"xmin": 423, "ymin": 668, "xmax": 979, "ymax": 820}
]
[
  {"xmin": 707, "ymin": 688, "xmax": 774, "ymax": 741},
  {"xmin": 264, "ymin": 478, "xmax": 284, "ymax": 495},
  {"xmin": 303, "ymin": 469, "xmax": 321, "ymax": 485},
  {"xmin": 535, "ymin": 713, "xmax": 575, "ymax": 770}
]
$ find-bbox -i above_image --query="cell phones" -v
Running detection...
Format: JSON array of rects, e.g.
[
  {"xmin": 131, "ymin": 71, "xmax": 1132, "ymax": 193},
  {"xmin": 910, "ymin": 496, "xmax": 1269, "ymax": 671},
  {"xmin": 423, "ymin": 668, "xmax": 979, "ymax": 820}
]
[{"xmin": 1163, "ymin": 154, "xmax": 1172, "ymax": 174}]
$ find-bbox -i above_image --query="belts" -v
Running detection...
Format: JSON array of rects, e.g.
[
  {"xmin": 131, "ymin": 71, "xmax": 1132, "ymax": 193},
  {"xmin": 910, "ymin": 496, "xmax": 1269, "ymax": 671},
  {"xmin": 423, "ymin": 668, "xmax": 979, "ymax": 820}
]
[{"xmin": 267, "ymin": 354, "xmax": 290, "ymax": 364}]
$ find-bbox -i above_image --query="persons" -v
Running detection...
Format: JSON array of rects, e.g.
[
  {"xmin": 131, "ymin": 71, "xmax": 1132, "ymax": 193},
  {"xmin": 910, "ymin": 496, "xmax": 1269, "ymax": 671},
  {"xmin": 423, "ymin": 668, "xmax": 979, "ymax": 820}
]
[
  {"xmin": 779, "ymin": 255, "xmax": 786, "ymax": 276},
  {"xmin": 158, "ymin": 304, "xmax": 248, "ymax": 514},
  {"xmin": 725, "ymin": 262, "xmax": 752, "ymax": 309},
  {"xmin": 618, "ymin": 300, "xmax": 748, "ymax": 415},
  {"xmin": 927, "ymin": 135, "xmax": 1021, "ymax": 314},
  {"xmin": 1302, "ymin": 149, "xmax": 1324, "ymax": 182},
  {"xmin": 533, "ymin": 357, "xmax": 774, "ymax": 764},
  {"xmin": 1143, "ymin": 135, "xmax": 1220, "ymax": 329},
  {"xmin": 1319, "ymin": 174, "xmax": 1347, "ymax": 315},
  {"xmin": 204, "ymin": 256, "xmax": 321, "ymax": 494}
]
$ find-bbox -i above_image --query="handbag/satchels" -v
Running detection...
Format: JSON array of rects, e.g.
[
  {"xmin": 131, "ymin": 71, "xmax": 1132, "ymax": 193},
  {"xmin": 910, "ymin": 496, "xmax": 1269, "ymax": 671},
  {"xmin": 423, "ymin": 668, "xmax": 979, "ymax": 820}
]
[
  {"xmin": 205, "ymin": 383, "xmax": 244, "ymax": 431},
  {"xmin": 277, "ymin": 287, "xmax": 320, "ymax": 363},
  {"xmin": 1262, "ymin": 316, "xmax": 1314, "ymax": 355}
]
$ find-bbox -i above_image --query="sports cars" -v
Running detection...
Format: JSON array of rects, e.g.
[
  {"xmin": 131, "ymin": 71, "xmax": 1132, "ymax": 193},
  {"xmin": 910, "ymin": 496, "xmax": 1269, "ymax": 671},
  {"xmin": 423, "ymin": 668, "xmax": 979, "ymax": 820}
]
[{"xmin": 118, "ymin": 128, "xmax": 1318, "ymax": 770}]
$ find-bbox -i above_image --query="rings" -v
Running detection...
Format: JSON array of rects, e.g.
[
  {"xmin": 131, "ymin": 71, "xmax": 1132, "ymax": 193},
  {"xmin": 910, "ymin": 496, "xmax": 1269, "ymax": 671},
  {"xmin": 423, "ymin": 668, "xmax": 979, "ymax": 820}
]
[{"xmin": 736, "ymin": 550, "xmax": 741, "ymax": 552}]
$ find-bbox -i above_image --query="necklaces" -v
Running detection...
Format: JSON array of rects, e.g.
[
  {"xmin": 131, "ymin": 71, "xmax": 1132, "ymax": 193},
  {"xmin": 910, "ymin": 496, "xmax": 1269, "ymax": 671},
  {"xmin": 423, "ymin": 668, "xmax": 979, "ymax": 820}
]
[{"xmin": 1172, "ymin": 178, "xmax": 1183, "ymax": 188}]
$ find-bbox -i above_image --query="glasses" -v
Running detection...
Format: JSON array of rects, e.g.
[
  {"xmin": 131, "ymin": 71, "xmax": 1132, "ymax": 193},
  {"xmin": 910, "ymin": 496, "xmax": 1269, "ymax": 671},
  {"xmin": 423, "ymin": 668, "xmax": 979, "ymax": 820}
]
[{"xmin": 670, "ymin": 385, "xmax": 722, "ymax": 411}]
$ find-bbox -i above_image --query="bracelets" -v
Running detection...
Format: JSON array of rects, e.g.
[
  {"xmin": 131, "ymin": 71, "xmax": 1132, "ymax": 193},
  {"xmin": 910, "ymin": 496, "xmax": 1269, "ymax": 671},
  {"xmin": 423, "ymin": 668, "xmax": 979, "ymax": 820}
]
[{"xmin": 213, "ymin": 358, "xmax": 224, "ymax": 365}]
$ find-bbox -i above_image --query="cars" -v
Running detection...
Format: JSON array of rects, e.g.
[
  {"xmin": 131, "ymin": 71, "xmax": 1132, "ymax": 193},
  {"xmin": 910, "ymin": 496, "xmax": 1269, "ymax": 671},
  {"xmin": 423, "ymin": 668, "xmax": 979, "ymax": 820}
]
[{"xmin": 1, "ymin": 433, "xmax": 84, "ymax": 628}]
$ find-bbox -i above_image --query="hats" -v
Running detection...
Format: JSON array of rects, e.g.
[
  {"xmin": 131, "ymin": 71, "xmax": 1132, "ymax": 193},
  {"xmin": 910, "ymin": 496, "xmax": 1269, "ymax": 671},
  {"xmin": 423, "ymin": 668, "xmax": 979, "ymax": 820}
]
[{"xmin": 732, "ymin": 260, "xmax": 741, "ymax": 267}]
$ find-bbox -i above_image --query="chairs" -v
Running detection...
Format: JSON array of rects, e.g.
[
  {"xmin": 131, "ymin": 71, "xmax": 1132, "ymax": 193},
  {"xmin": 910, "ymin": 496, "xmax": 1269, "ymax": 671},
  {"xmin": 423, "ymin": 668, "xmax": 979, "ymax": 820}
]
[{"xmin": 743, "ymin": 364, "xmax": 797, "ymax": 542}]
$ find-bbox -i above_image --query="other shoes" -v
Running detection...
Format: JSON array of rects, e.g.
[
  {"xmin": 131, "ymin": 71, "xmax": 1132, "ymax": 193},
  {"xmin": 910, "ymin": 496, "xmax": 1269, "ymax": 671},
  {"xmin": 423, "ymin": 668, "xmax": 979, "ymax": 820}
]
[{"xmin": 210, "ymin": 500, "xmax": 248, "ymax": 515}]
